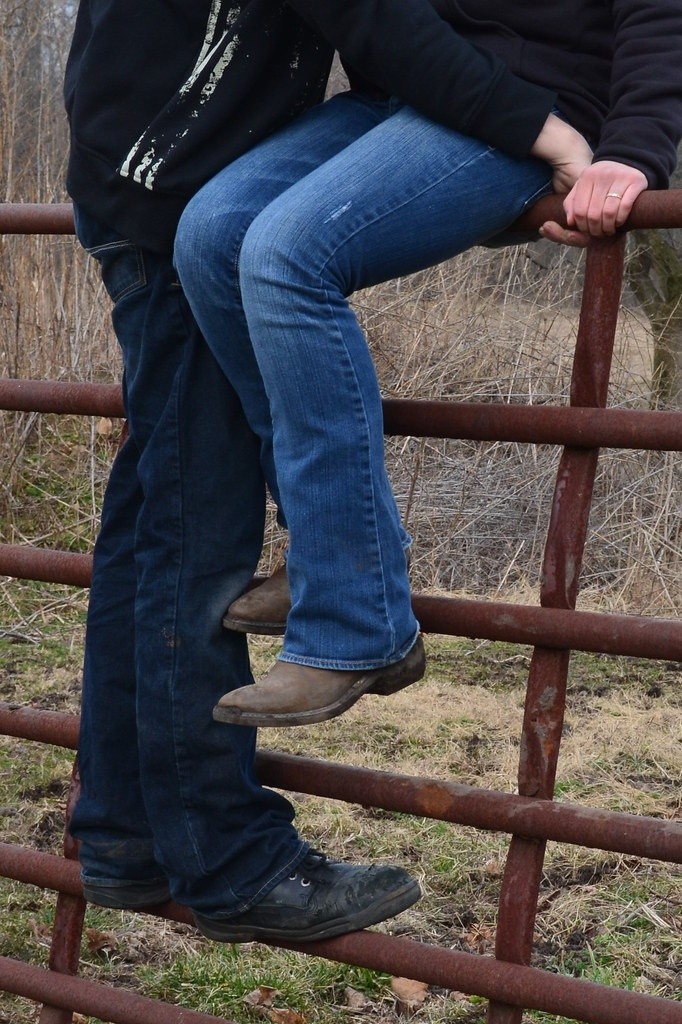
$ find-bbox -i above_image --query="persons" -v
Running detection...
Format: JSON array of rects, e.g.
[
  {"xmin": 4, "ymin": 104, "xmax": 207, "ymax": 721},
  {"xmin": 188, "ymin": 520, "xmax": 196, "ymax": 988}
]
[
  {"xmin": 64, "ymin": 1, "xmax": 595, "ymax": 944},
  {"xmin": 172, "ymin": 1, "xmax": 681, "ymax": 727}
]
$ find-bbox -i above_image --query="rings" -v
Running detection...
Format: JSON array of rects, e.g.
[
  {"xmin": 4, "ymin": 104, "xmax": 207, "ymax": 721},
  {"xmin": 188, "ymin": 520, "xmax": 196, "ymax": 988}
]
[{"xmin": 606, "ymin": 192, "xmax": 622, "ymax": 200}]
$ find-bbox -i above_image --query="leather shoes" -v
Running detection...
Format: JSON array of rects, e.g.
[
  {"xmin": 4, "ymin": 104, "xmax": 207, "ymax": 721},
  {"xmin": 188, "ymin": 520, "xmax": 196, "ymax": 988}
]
[
  {"xmin": 213, "ymin": 639, "xmax": 426, "ymax": 727},
  {"xmin": 224, "ymin": 546, "xmax": 410, "ymax": 635},
  {"xmin": 84, "ymin": 789, "xmax": 421, "ymax": 945}
]
